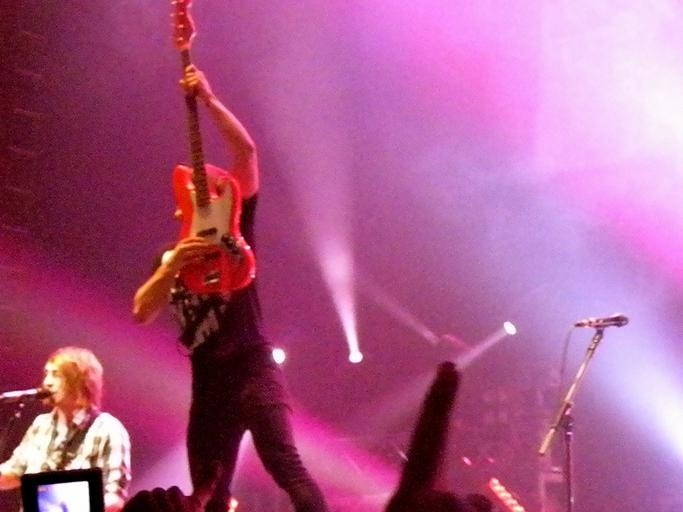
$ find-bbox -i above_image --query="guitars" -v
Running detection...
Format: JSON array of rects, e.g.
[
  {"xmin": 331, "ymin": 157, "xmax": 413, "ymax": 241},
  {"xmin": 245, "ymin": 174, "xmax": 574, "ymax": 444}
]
[{"xmin": 169, "ymin": 1, "xmax": 258, "ymax": 298}]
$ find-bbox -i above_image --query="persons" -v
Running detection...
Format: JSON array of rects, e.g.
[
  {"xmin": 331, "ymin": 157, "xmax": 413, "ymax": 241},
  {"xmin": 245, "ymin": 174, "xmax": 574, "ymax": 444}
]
[
  {"xmin": 130, "ymin": 62, "xmax": 331, "ymax": 510},
  {"xmin": 381, "ymin": 357, "xmax": 494, "ymax": 512},
  {"xmin": 0, "ymin": 346, "xmax": 134, "ymax": 512},
  {"xmin": 121, "ymin": 459, "xmax": 226, "ymax": 510}
]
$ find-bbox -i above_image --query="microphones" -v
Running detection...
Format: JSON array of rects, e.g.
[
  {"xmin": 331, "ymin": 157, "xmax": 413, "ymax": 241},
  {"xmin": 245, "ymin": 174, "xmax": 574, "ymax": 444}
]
[
  {"xmin": 0, "ymin": 387, "xmax": 51, "ymax": 404},
  {"xmin": 575, "ymin": 315, "xmax": 628, "ymax": 327}
]
